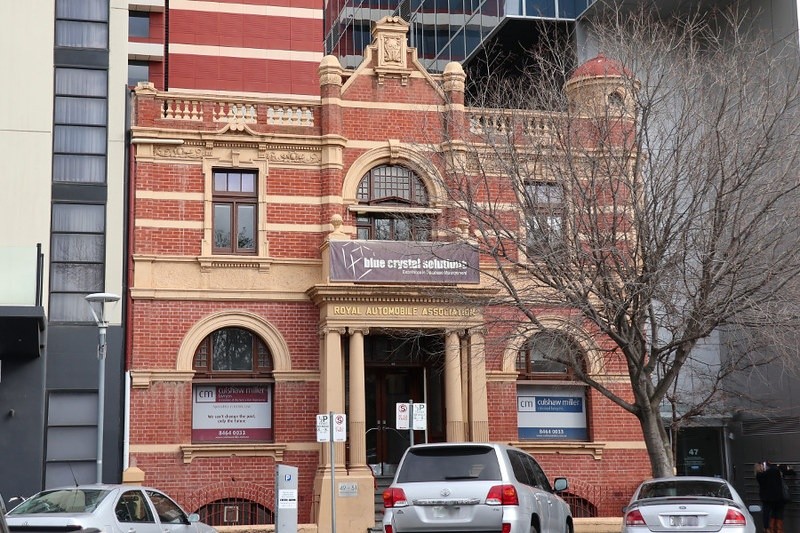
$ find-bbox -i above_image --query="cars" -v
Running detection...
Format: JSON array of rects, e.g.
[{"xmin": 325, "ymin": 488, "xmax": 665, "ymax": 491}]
[
  {"xmin": 620, "ymin": 475, "xmax": 757, "ymax": 533},
  {"xmin": 3, "ymin": 483, "xmax": 220, "ymax": 533}
]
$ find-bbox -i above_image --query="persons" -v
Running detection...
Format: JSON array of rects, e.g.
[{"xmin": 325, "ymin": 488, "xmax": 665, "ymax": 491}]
[{"xmin": 754, "ymin": 461, "xmax": 794, "ymax": 533}]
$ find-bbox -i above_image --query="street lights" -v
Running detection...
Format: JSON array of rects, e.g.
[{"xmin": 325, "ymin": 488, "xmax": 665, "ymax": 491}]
[{"xmin": 84, "ymin": 292, "xmax": 121, "ymax": 485}]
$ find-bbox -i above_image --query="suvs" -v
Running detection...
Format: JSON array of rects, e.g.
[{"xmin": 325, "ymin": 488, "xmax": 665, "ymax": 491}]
[{"xmin": 383, "ymin": 441, "xmax": 575, "ymax": 533}]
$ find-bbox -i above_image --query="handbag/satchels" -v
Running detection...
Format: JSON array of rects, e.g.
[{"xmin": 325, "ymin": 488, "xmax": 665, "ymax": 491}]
[{"xmin": 777, "ymin": 467, "xmax": 793, "ymax": 505}]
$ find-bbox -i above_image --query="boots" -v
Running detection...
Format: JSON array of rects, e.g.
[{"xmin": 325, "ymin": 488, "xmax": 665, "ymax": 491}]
[
  {"xmin": 776, "ymin": 518, "xmax": 784, "ymax": 533},
  {"xmin": 763, "ymin": 518, "xmax": 775, "ymax": 533}
]
